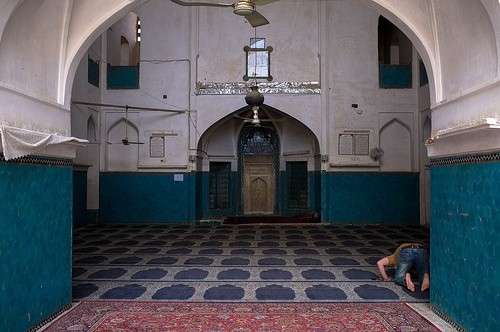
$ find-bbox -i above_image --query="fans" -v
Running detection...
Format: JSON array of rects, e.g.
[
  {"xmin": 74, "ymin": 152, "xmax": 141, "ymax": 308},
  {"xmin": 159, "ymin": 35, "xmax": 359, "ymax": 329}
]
[
  {"xmin": 107, "ymin": 108, "xmax": 145, "ymax": 145},
  {"xmin": 235, "ymin": 106, "xmax": 286, "ymax": 124},
  {"xmin": 172, "ymin": 0, "xmax": 276, "ymax": 27}
]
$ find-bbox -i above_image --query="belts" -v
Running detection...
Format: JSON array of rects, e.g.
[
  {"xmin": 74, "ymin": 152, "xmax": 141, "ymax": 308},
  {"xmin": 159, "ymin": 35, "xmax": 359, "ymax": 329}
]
[{"xmin": 401, "ymin": 245, "xmax": 424, "ymax": 250}]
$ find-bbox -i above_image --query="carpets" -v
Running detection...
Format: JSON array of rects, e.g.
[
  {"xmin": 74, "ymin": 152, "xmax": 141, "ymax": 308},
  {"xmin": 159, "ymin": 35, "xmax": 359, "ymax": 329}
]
[{"xmin": 40, "ymin": 299, "xmax": 468, "ymax": 332}]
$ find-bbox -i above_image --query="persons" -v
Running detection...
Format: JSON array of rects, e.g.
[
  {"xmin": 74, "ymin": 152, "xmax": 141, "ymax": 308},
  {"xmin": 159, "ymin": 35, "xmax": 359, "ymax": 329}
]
[{"xmin": 377, "ymin": 243, "xmax": 430, "ymax": 292}]
